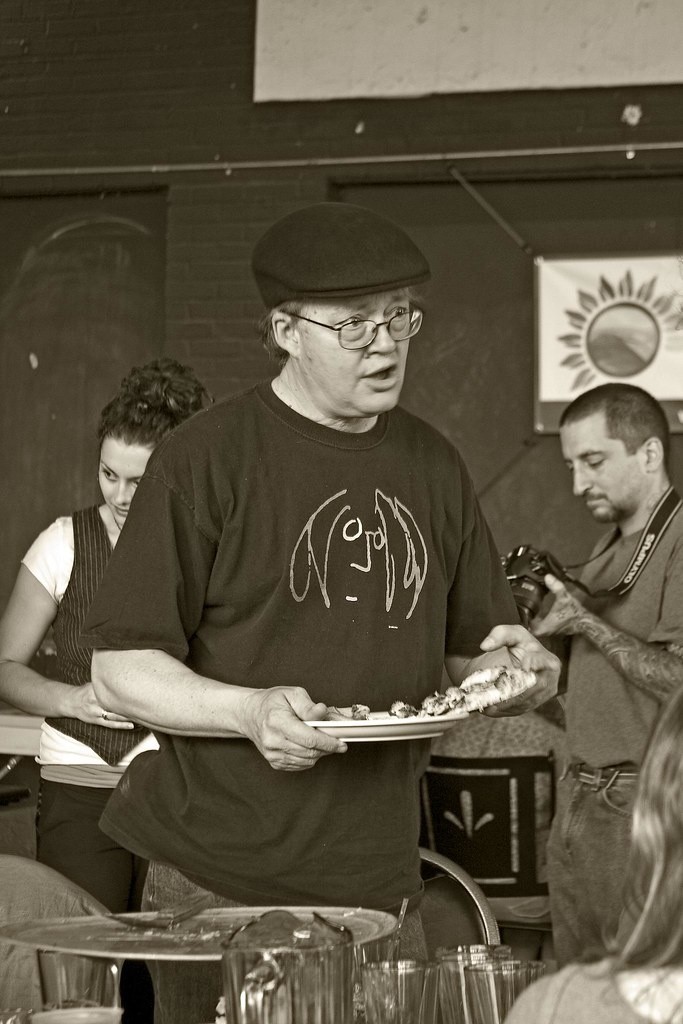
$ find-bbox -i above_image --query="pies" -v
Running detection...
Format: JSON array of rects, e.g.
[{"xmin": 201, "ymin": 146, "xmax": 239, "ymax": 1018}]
[{"xmin": 323, "ymin": 664, "xmax": 537, "ymax": 721}]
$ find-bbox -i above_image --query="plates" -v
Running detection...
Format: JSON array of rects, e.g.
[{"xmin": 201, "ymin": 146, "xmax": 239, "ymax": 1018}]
[{"xmin": 301, "ymin": 709, "xmax": 470, "ymax": 742}]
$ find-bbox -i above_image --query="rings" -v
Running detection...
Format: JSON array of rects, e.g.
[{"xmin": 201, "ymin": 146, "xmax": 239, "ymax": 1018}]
[{"xmin": 100, "ymin": 709, "xmax": 108, "ymax": 718}]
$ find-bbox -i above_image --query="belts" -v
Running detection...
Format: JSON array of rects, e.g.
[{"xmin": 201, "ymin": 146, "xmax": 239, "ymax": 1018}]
[{"xmin": 573, "ymin": 766, "xmax": 637, "ymax": 791}]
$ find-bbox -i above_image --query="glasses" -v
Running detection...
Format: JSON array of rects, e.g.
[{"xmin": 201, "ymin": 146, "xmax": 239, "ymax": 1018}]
[{"xmin": 280, "ymin": 301, "xmax": 425, "ymax": 352}]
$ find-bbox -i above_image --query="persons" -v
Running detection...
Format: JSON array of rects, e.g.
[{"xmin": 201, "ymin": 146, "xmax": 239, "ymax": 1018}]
[
  {"xmin": 1, "ymin": 357, "xmax": 223, "ymax": 1023},
  {"xmin": 82, "ymin": 196, "xmax": 563, "ymax": 1024},
  {"xmin": 495, "ymin": 380, "xmax": 683, "ymax": 1024}
]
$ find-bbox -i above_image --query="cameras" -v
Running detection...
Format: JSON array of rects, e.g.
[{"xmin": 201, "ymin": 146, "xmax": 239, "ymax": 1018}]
[{"xmin": 502, "ymin": 544, "xmax": 565, "ymax": 628}]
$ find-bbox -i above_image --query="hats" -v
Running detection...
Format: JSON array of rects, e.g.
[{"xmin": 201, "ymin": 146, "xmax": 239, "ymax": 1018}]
[{"xmin": 251, "ymin": 203, "xmax": 431, "ymax": 311}]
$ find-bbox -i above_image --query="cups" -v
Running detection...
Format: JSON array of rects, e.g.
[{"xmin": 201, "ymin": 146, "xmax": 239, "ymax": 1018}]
[
  {"xmin": 1, "ymin": 949, "xmax": 124, "ymax": 1024},
  {"xmin": 222, "ymin": 912, "xmax": 550, "ymax": 1024}
]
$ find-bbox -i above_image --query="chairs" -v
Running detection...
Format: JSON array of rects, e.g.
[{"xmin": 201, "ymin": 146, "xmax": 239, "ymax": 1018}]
[{"xmin": 407, "ymin": 751, "xmax": 560, "ymax": 895}]
